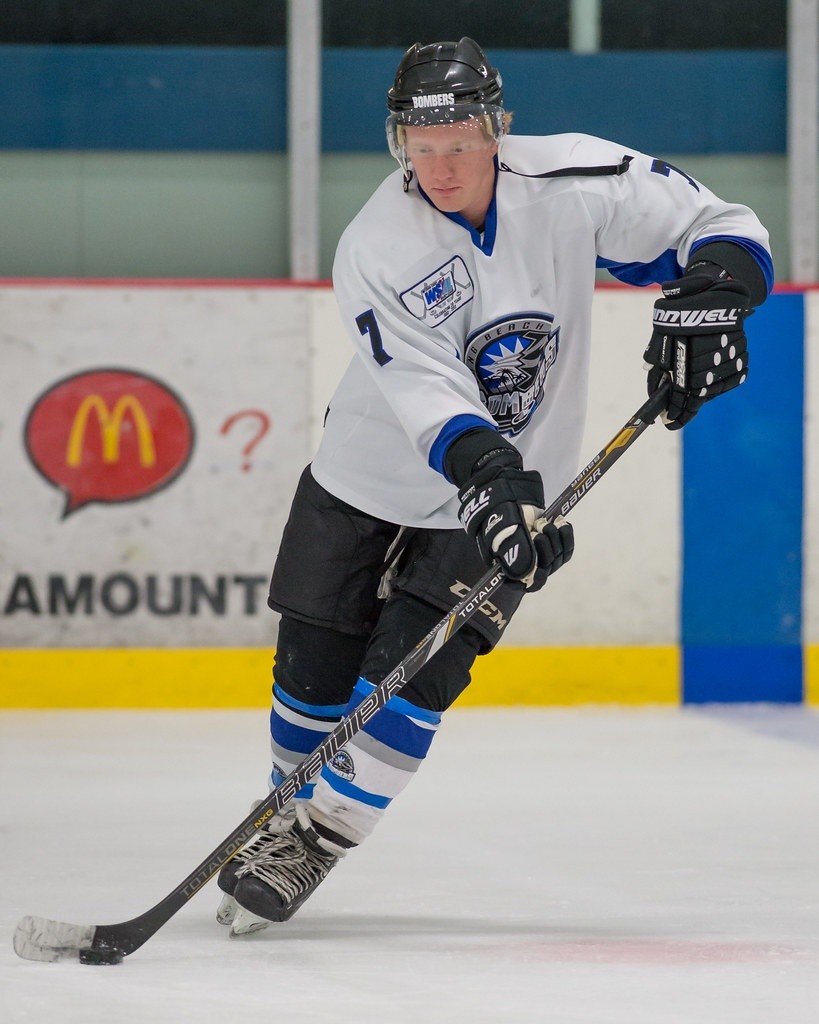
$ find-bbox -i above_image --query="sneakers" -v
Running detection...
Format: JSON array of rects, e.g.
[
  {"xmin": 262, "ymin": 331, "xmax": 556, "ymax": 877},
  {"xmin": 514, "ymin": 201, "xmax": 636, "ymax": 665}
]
[{"xmin": 216, "ymin": 802, "xmax": 363, "ymax": 937}]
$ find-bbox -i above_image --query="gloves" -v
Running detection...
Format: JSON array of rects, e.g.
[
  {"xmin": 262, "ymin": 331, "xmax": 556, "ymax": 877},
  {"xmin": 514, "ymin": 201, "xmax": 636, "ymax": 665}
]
[
  {"xmin": 644, "ymin": 272, "xmax": 755, "ymax": 431},
  {"xmin": 458, "ymin": 464, "xmax": 574, "ymax": 592}
]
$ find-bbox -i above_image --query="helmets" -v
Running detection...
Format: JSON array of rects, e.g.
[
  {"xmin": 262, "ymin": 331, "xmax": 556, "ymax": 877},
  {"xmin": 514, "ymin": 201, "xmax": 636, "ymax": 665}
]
[{"xmin": 386, "ymin": 36, "xmax": 503, "ymax": 147}]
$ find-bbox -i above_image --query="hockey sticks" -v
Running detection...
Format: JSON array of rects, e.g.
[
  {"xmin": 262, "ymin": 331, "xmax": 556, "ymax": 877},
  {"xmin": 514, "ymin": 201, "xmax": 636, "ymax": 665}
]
[{"xmin": 11, "ymin": 383, "xmax": 674, "ymax": 969}]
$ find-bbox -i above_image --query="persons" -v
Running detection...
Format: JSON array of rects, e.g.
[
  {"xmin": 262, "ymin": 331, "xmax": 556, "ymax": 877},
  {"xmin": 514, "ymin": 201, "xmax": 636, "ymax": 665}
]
[{"xmin": 216, "ymin": 37, "xmax": 776, "ymax": 940}]
